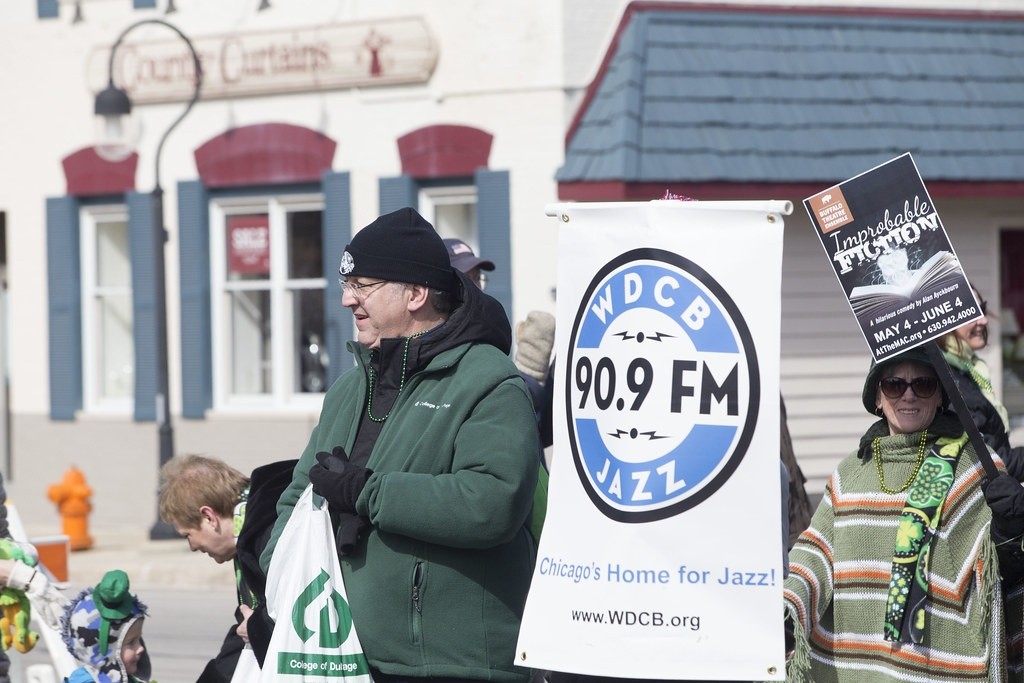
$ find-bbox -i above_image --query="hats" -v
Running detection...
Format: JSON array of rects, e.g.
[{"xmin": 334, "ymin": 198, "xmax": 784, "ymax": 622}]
[
  {"xmin": 337, "ymin": 207, "xmax": 464, "ymax": 295},
  {"xmin": 863, "ymin": 347, "xmax": 950, "ymax": 417},
  {"xmin": 443, "ymin": 238, "xmax": 496, "ymax": 273}
]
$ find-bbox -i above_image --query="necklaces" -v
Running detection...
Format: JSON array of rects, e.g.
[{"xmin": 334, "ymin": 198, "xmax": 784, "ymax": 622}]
[
  {"xmin": 366, "ymin": 330, "xmax": 422, "ymax": 422},
  {"xmin": 965, "ymin": 358, "xmax": 993, "ymax": 391},
  {"xmin": 868, "ymin": 431, "xmax": 926, "ymax": 492}
]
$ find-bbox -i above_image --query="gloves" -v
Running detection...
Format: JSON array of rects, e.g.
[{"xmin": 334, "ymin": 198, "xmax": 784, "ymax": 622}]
[
  {"xmin": 981, "ymin": 471, "xmax": 1024, "ymax": 538},
  {"xmin": 329, "ymin": 503, "xmax": 374, "ymax": 558},
  {"xmin": 785, "ymin": 610, "xmax": 796, "ymax": 653},
  {"xmin": 310, "ymin": 444, "xmax": 373, "ymax": 511},
  {"xmin": 5, "ymin": 560, "xmax": 73, "ymax": 634}
]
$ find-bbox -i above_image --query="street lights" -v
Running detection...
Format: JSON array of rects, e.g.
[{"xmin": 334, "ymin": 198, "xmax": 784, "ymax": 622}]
[{"xmin": 91, "ymin": 17, "xmax": 186, "ymax": 540}]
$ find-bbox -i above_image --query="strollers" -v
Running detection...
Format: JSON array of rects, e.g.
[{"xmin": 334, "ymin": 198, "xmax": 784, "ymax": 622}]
[{"xmin": 25, "ymin": 566, "xmax": 77, "ymax": 683}]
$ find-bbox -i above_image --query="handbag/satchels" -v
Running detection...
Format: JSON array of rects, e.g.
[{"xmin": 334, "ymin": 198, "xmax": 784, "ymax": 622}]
[{"xmin": 261, "ymin": 483, "xmax": 377, "ymax": 683}]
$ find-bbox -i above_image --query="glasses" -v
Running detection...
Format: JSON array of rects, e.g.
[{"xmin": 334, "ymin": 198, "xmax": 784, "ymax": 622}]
[
  {"xmin": 338, "ymin": 281, "xmax": 389, "ymax": 297},
  {"xmin": 877, "ymin": 376, "xmax": 939, "ymax": 399},
  {"xmin": 468, "ymin": 274, "xmax": 489, "ymax": 291}
]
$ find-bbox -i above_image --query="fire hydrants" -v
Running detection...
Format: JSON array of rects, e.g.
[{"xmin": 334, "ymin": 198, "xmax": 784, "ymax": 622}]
[{"xmin": 48, "ymin": 465, "xmax": 92, "ymax": 553}]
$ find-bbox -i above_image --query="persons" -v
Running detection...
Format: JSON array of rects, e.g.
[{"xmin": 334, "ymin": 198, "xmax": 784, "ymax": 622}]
[
  {"xmin": 787, "ymin": 348, "xmax": 1024, "ymax": 683},
  {"xmin": 0, "ymin": 472, "xmax": 72, "ymax": 683},
  {"xmin": 59, "ymin": 570, "xmax": 154, "ymax": 683},
  {"xmin": 437, "ymin": 232, "xmax": 495, "ymax": 294},
  {"xmin": 157, "ymin": 450, "xmax": 298, "ymax": 683},
  {"xmin": 937, "ymin": 281, "xmax": 1024, "ymax": 484},
  {"xmin": 511, "ymin": 307, "xmax": 813, "ymax": 683},
  {"xmin": 258, "ymin": 210, "xmax": 542, "ymax": 683}
]
